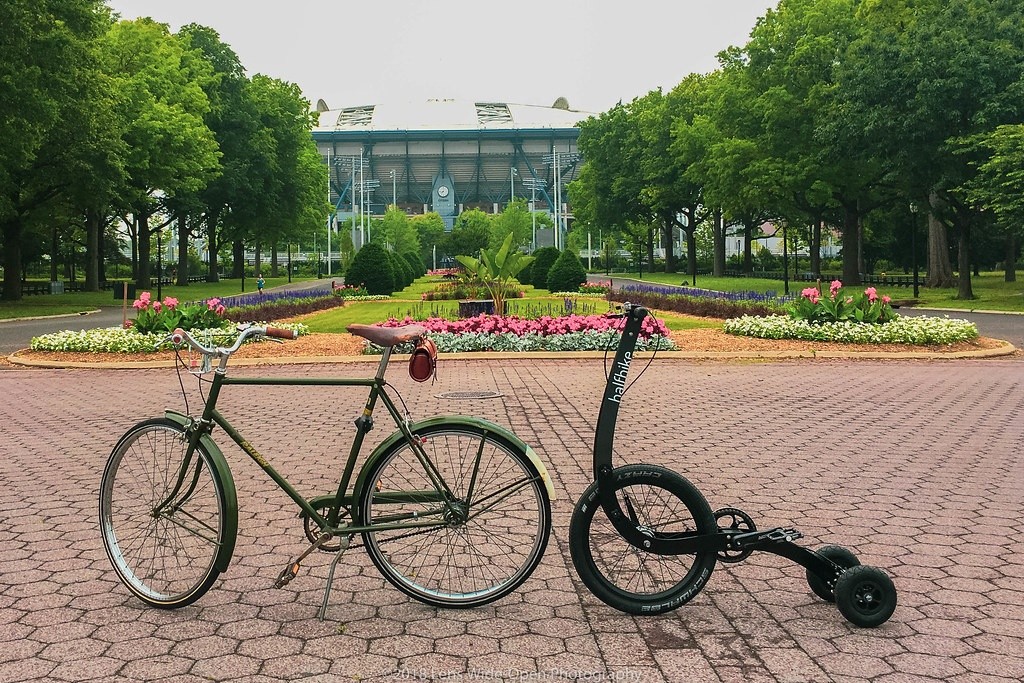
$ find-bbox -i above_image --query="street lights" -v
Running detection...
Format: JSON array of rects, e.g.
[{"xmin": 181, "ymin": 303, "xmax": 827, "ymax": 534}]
[
  {"xmin": 509, "ymin": 167, "xmax": 518, "ymax": 205},
  {"xmin": 779, "ymin": 218, "xmax": 791, "ymax": 295},
  {"xmin": 909, "ymin": 201, "xmax": 921, "ymax": 297},
  {"xmin": 637, "ymin": 235, "xmax": 643, "ymax": 278},
  {"xmin": 691, "ymin": 230, "xmax": 698, "ymax": 286},
  {"xmin": 241, "ymin": 238, "xmax": 246, "ymax": 292},
  {"xmin": 317, "ymin": 244, "xmax": 322, "ymax": 274},
  {"xmin": 156, "ymin": 230, "xmax": 164, "ymax": 302},
  {"xmin": 389, "ymin": 169, "xmax": 397, "ymax": 218}
]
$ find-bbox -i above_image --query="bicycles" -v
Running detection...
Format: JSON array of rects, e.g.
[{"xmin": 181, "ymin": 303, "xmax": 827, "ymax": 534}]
[{"xmin": 97, "ymin": 322, "xmax": 559, "ymax": 623}]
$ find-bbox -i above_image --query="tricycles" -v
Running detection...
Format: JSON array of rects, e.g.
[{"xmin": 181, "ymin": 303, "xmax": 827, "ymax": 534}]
[{"xmin": 566, "ymin": 300, "xmax": 897, "ymax": 629}]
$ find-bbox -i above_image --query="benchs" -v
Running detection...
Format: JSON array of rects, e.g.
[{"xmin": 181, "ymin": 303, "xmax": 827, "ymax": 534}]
[
  {"xmin": 747, "ymin": 272, "xmax": 790, "ymax": 281},
  {"xmin": 697, "ymin": 269, "xmax": 709, "ymax": 276},
  {"xmin": 220, "ymin": 273, "xmax": 232, "ymax": 280},
  {"xmin": 151, "ymin": 278, "xmax": 173, "ymax": 286},
  {"xmin": 99, "ymin": 280, "xmax": 127, "ymax": 291},
  {"xmin": 187, "ymin": 275, "xmax": 208, "ymax": 283},
  {"xmin": 860, "ymin": 275, "xmax": 930, "ymax": 288},
  {"xmin": 721, "ymin": 270, "xmax": 746, "ymax": 278},
  {"xmin": 22, "ymin": 283, "xmax": 50, "ymax": 295},
  {"xmin": 793, "ymin": 273, "xmax": 843, "ymax": 284},
  {"xmin": 64, "ymin": 281, "xmax": 85, "ymax": 293}
]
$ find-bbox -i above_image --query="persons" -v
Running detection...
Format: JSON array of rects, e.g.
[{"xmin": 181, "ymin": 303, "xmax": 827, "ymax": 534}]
[{"xmin": 256, "ymin": 274, "xmax": 265, "ymax": 295}]
